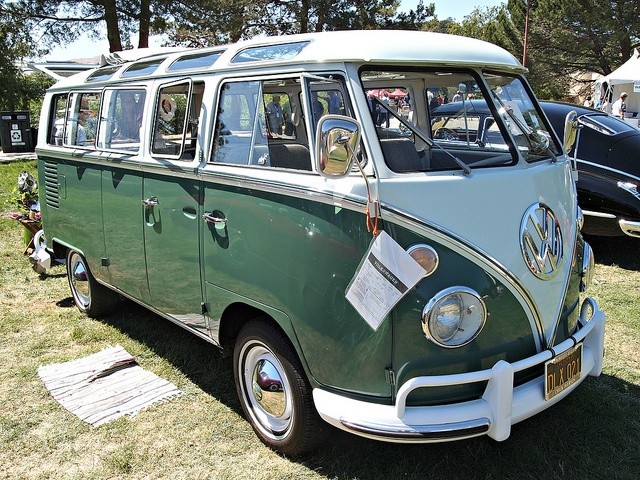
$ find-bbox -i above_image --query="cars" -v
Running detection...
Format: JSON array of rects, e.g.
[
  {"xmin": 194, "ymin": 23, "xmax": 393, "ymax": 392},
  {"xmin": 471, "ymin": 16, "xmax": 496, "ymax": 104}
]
[{"xmin": 374, "ymin": 96, "xmax": 640, "ymax": 241}]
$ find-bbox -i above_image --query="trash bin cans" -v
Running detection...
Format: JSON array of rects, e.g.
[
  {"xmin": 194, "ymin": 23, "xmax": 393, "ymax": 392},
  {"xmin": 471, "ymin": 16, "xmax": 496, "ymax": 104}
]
[{"xmin": 0, "ymin": 112, "xmax": 31, "ymax": 153}]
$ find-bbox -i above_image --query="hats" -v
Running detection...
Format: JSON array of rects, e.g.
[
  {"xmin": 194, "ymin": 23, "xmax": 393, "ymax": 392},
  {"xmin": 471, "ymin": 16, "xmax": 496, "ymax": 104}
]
[
  {"xmin": 159, "ymin": 94, "xmax": 177, "ymax": 122},
  {"xmin": 272, "ymin": 93, "xmax": 281, "ymax": 97},
  {"xmin": 58, "ymin": 97, "xmax": 92, "ymax": 112}
]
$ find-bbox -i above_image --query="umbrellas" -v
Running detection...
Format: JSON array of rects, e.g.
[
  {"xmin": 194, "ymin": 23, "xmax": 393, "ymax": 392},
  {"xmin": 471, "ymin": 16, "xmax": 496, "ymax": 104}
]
[{"xmin": 389, "ymin": 89, "xmax": 408, "ymax": 99}]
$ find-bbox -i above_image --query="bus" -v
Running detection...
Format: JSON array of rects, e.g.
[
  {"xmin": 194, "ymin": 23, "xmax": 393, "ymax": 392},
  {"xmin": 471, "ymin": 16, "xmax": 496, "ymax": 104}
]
[{"xmin": 25, "ymin": 29, "xmax": 608, "ymax": 459}]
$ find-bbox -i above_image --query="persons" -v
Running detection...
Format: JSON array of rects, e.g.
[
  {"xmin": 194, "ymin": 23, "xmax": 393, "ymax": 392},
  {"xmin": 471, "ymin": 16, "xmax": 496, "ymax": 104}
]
[
  {"xmin": 311, "ymin": 92, "xmax": 324, "ymax": 124},
  {"xmin": 377, "ymin": 92, "xmax": 390, "ymax": 126},
  {"xmin": 427, "ymin": 89, "xmax": 448, "ymax": 116},
  {"xmin": 397, "ymin": 96, "xmax": 407, "ymax": 112},
  {"xmin": 467, "ymin": 90, "xmax": 476, "ymax": 121},
  {"xmin": 367, "ymin": 94, "xmax": 379, "ymax": 126},
  {"xmin": 584, "ymin": 96, "xmax": 611, "ymax": 114},
  {"xmin": 611, "ymin": 92, "xmax": 628, "ymax": 116},
  {"xmin": 51, "ymin": 97, "xmax": 91, "ymax": 145},
  {"xmin": 317, "ymin": 91, "xmax": 341, "ymax": 114},
  {"xmin": 265, "ymin": 93, "xmax": 284, "ymax": 135},
  {"xmin": 452, "ymin": 90, "xmax": 463, "ymax": 119},
  {"xmin": 120, "ymin": 93, "xmax": 140, "ymax": 140}
]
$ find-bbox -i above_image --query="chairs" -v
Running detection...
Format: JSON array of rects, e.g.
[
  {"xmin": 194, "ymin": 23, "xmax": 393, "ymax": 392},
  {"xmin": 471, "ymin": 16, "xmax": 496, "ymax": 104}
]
[
  {"xmin": 381, "ymin": 135, "xmax": 424, "ymax": 173},
  {"xmin": 223, "ymin": 142, "xmax": 312, "ymax": 171}
]
